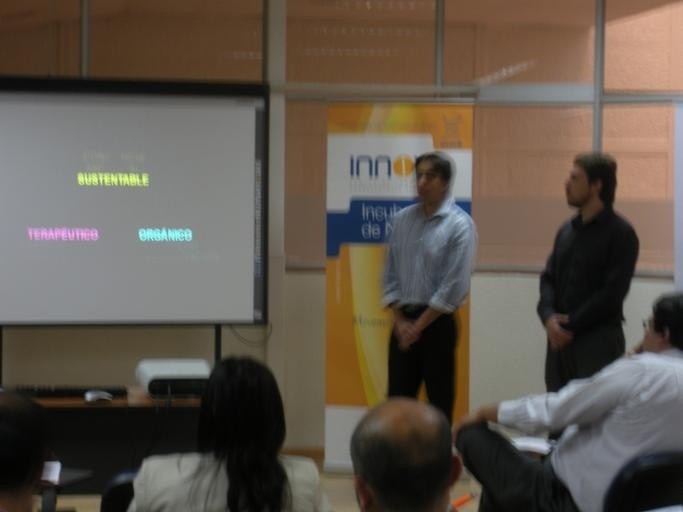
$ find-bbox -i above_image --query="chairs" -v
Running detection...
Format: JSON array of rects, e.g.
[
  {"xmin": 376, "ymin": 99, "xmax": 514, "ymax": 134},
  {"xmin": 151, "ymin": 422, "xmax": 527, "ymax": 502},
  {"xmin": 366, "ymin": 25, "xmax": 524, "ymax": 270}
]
[
  {"xmin": 100, "ymin": 471, "xmax": 135, "ymax": 512},
  {"xmin": 601, "ymin": 449, "xmax": 683, "ymax": 512}
]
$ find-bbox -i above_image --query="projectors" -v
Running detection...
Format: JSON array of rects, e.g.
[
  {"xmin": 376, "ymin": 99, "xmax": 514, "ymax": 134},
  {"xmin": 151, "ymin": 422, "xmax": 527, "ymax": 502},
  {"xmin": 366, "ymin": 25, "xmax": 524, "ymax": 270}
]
[{"xmin": 134, "ymin": 355, "xmax": 213, "ymax": 400}]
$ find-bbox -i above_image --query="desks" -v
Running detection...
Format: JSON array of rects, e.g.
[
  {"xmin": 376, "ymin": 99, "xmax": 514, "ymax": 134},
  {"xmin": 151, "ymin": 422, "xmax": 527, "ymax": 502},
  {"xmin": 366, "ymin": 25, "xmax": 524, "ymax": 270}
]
[{"xmin": 0, "ymin": 382, "xmax": 203, "ymax": 480}]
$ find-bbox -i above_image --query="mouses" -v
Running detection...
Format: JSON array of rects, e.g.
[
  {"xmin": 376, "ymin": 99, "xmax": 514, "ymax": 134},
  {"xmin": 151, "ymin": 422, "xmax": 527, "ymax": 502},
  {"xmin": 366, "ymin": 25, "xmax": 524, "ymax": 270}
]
[{"xmin": 85, "ymin": 389, "xmax": 110, "ymax": 404}]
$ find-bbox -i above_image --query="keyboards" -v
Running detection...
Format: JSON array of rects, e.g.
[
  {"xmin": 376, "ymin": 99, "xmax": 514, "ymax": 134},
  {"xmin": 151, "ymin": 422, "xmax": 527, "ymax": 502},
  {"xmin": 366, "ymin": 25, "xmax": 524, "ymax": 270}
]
[{"xmin": 14, "ymin": 384, "xmax": 129, "ymax": 398}]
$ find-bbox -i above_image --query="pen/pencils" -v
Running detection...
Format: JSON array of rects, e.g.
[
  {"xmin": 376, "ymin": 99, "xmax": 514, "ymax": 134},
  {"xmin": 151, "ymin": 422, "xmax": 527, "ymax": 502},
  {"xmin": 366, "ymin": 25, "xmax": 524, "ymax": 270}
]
[{"xmin": 451, "ymin": 495, "xmax": 476, "ymax": 509}]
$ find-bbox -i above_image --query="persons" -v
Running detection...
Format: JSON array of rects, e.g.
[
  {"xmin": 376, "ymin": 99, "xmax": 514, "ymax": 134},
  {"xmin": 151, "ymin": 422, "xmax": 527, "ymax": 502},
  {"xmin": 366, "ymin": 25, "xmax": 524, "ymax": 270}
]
[
  {"xmin": 124, "ymin": 354, "xmax": 325, "ymax": 512},
  {"xmin": 347, "ymin": 393, "xmax": 463, "ymax": 512},
  {"xmin": 536, "ymin": 151, "xmax": 640, "ymax": 441},
  {"xmin": 455, "ymin": 288, "xmax": 683, "ymax": 512},
  {"xmin": 1, "ymin": 391, "xmax": 50, "ymax": 512},
  {"xmin": 380, "ymin": 152, "xmax": 473, "ymax": 432}
]
